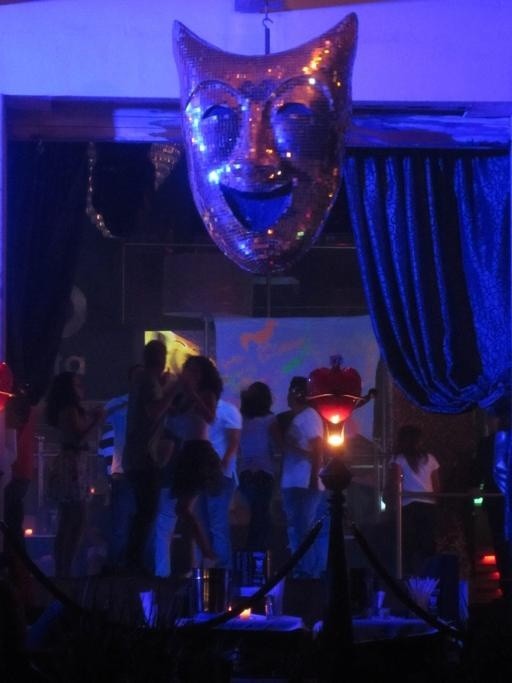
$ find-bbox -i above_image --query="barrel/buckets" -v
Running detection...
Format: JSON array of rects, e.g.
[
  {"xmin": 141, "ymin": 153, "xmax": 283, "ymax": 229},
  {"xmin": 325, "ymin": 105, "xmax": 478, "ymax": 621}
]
[{"xmin": 192, "ymin": 567, "xmax": 231, "ymax": 614}]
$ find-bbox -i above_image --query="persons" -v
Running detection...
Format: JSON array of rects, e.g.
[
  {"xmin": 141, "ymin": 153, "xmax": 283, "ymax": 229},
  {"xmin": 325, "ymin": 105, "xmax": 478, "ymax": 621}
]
[{"xmin": 0, "ymin": 338, "xmax": 512, "ymax": 683}]
